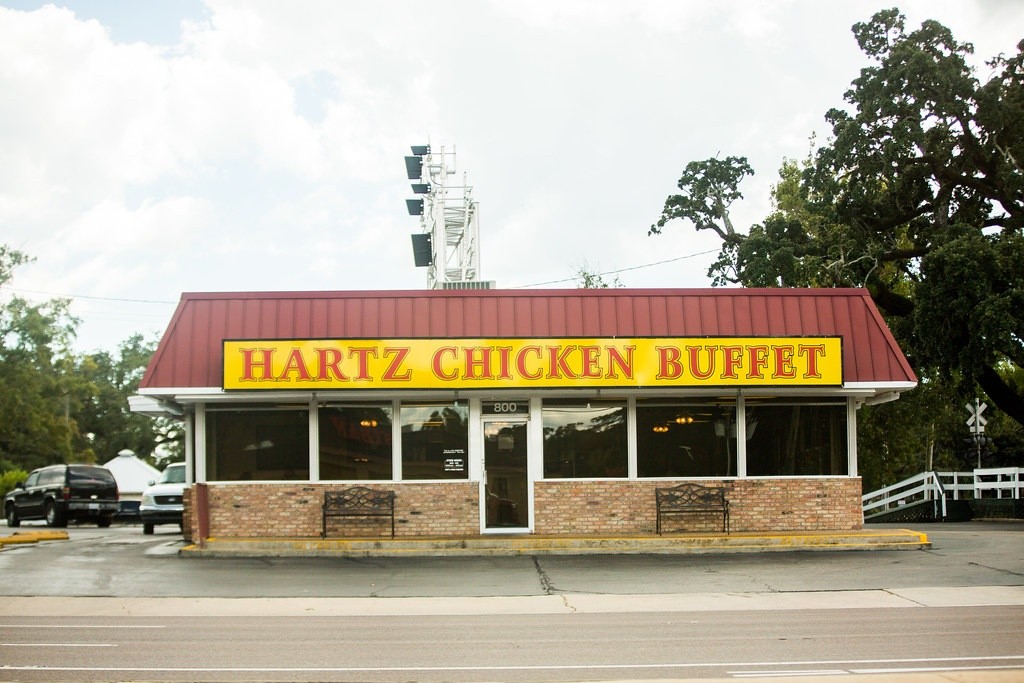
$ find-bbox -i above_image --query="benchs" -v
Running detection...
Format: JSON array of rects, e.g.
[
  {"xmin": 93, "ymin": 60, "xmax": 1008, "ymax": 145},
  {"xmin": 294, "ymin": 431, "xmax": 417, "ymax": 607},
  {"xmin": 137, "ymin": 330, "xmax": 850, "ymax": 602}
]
[
  {"xmin": 321, "ymin": 486, "xmax": 396, "ymax": 539},
  {"xmin": 655, "ymin": 482, "xmax": 731, "ymax": 534}
]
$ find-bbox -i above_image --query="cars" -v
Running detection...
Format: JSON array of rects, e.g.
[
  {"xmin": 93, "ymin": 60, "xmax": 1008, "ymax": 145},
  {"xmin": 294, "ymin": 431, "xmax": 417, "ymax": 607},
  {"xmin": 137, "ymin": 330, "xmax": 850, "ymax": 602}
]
[{"xmin": 487, "ymin": 490, "xmax": 518, "ymax": 524}]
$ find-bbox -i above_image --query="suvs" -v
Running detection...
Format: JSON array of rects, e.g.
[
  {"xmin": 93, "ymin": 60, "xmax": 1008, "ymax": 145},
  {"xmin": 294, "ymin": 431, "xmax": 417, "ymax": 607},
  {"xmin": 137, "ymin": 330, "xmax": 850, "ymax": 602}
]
[
  {"xmin": 4, "ymin": 463, "xmax": 122, "ymax": 528},
  {"xmin": 137, "ymin": 462, "xmax": 186, "ymax": 533}
]
